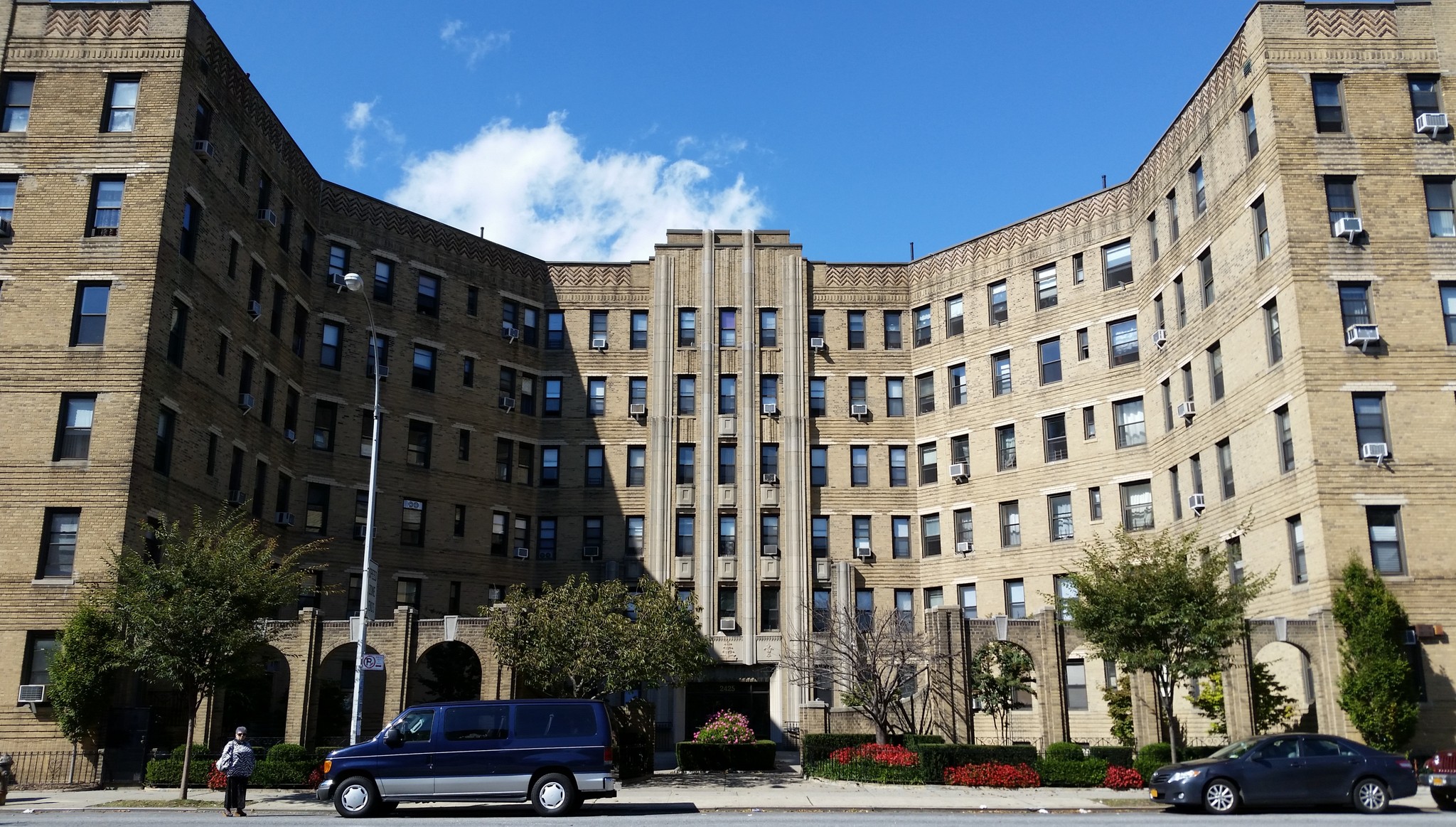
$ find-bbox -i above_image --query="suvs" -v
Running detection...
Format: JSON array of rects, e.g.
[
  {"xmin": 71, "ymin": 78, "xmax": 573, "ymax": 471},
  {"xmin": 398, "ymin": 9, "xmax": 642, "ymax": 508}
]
[{"xmin": 1420, "ymin": 746, "xmax": 1456, "ymax": 807}]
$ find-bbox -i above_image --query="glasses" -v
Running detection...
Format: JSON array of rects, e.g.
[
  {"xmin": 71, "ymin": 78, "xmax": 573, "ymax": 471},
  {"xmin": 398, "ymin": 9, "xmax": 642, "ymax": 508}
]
[{"xmin": 237, "ymin": 733, "xmax": 247, "ymax": 736}]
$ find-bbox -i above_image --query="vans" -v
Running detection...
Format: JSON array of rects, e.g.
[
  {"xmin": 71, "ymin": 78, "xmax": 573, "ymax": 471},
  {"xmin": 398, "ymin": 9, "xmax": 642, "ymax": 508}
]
[{"xmin": 313, "ymin": 701, "xmax": 622, "ymax": 818}]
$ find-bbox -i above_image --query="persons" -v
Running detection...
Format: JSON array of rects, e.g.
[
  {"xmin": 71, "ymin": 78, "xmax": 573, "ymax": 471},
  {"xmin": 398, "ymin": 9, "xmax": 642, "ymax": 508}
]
[{"xmin": 222, "ymin": 727, "xmax": 255, "ymax": 817}]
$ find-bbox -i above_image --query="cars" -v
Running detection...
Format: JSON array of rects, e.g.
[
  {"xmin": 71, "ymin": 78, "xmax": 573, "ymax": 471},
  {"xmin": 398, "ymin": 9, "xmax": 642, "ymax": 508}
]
[{"xmin": 1144, "ymin": 731, "xmax": 1426, "ymax": 816}]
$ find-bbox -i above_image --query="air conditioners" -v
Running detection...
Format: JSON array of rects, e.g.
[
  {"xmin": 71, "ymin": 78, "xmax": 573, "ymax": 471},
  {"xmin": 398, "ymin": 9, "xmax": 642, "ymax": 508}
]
[
  {"xmin": 1345, "ymin": 323, "xmax": 1382, "ymax": 345},
  {"xmin": 957, "ymin": 542, "xmax": 972, "ymax": 552},
  {"xmin": 1333, "ymin": 216, "xmax": 1364, "ymax": 239},
  {"xmin": 631, "ymin": 404, "xmax": 645, "ymax": 414},
  {"xmin": 1189, "ymin": 493, "xmax": 1205, "ymax": 510},
  {"xmin": 764, "ymin": 544, "xmax": 777, "ymax": 555},
  {"xmin": 1177, "ymin": 401, "xmax": 1195, "ymax": 418},
  {"xmin": 238, "ymin": 393, "xmax": 254, "ymax": 410},
  {"xmin": 327, "ymin": 273, "xmax": 348, "ymax": 292},
  {"xmin": 764, "ymin": 403, "xmax": 776, "ymax": 413},
  {"xmin": 1402, "ymin": 630, "xmax": 1416, "ymax": 645},
  {"xmin": 275, "ymin": 512, "xmax": 296, "ymax": 526},
  {"xmin": 949, "ymin": 463, "xmax": 970, "ymax": 477},
  {"xmin": 503, "ymin": 327, "xmax": 519, "ymax": 339},
  {"xmin": 258, "ymin": 209, "xmax": 277, "ymax": 228},
  {"xmin": 592, "ymin": 338, "xmax": 605, "ymax": 348},
  {"xmin": 193, "ymin": 139, "xmax": 215, "ymax": 160},
  {"xmin": 347, "ymin": 610, "xmax": 359, "ymax": 620},
  {"xmin": 247, "ymin": 300, "xmax": 261, "ymax": 317},
  {"xmin": 499, "ymin": 397, "xmax": 515, "ymax": 408},
  {"xmin": 403, "ymin": 500, "xmax": 423, "ymax": 510},
  {"xmin": 284, "ymin": 429, "xmax": 295, "ymax": 441},
  {"xmin": 369, "ymin": 364, "xmax": 389, "ymax": 380},
  {"xmin": 1153, "ymin": 329, "xmax": 1165, "ymax": 344},
  {"xmin": 851, "ymin": 404, "xmax": 867, "ymax": 416},
  {"xmin": 584, "ymin": 546, "xmax": 599, "ymax": 557},
  {"xmin": 1416, "ymin": 111, "xmax": 1452, "ymax": 132},
  {"xmin": 811, "ymin": 338, "xmax": 824, "ymax": 348},
  {"xmin": 513, "ymin": 547, "xmax": 529, "ymax": 557},
  {"xmin": 355, "ymin": 524, "xmax": 377, "ymax": 538},
  {"xmin": 857, "ymin": 548, "xmax": 871, "ymax": 558},
  {"xmin": 227, "ymin": 489, "xmax": 246, "ymax": 506},
  {"xmin": 1365, "ymin": 441, "xmax": 1390, "ymax": 462},
  {"xmin": 763, "ymin": 473, "xmax": 776, "ymax": 483},
  {"xmin": 720, "ymin": 619, "xmax": 735, "ymax": 630},
  {"xmin": 17, "ymin": 682, "xmax": 51, "ymax": 704}
]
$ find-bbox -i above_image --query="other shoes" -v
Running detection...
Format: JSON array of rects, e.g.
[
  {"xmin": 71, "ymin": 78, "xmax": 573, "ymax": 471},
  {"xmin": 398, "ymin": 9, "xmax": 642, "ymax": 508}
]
[
  {"xmin": 236, "ymin": 810, "xmax": 247, "ymax": 816},
  {"xmin": 224, "ymin": 809, "xmax": 233, "ymax": 816}
]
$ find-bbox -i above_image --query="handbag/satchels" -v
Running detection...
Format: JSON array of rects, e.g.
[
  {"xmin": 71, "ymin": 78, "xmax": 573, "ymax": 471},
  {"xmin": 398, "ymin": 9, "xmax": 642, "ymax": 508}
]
[{"xmin": 216, "ymin": 758, "xmax": 226, "ymax": 772}]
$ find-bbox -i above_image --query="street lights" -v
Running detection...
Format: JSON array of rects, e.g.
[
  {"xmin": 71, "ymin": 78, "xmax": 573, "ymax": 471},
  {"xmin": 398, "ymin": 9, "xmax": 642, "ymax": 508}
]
[{"xmin": 340, "ymin": 264, "xmax": 394, "ymax": 741}]
